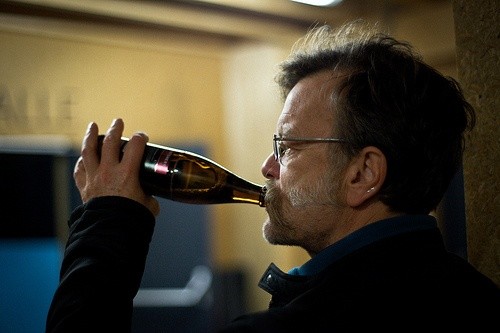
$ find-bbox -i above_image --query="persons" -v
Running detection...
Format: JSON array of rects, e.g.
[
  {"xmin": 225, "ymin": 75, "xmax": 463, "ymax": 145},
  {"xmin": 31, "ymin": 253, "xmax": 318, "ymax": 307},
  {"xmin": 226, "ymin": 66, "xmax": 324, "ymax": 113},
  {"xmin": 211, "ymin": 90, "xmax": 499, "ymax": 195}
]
[{"xmin": 46, "ymin": 19, "xmax": 500, "ymax": 333}]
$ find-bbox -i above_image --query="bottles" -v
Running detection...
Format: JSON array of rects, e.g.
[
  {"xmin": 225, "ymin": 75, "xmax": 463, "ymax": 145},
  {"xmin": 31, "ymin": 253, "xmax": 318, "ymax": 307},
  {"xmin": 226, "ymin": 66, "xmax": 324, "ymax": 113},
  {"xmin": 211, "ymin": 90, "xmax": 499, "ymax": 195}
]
[{"xmin": 96, "ymin": 134, "xmax": 266, "ymax": 207}]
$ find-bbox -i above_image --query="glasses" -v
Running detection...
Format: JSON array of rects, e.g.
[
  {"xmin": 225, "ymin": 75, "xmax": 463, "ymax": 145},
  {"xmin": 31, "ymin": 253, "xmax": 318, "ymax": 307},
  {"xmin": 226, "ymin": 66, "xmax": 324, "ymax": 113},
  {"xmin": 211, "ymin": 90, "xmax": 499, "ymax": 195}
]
[{"xmin": 273, "ymin": 135, "xmax": 345, "ymax": 161}]
